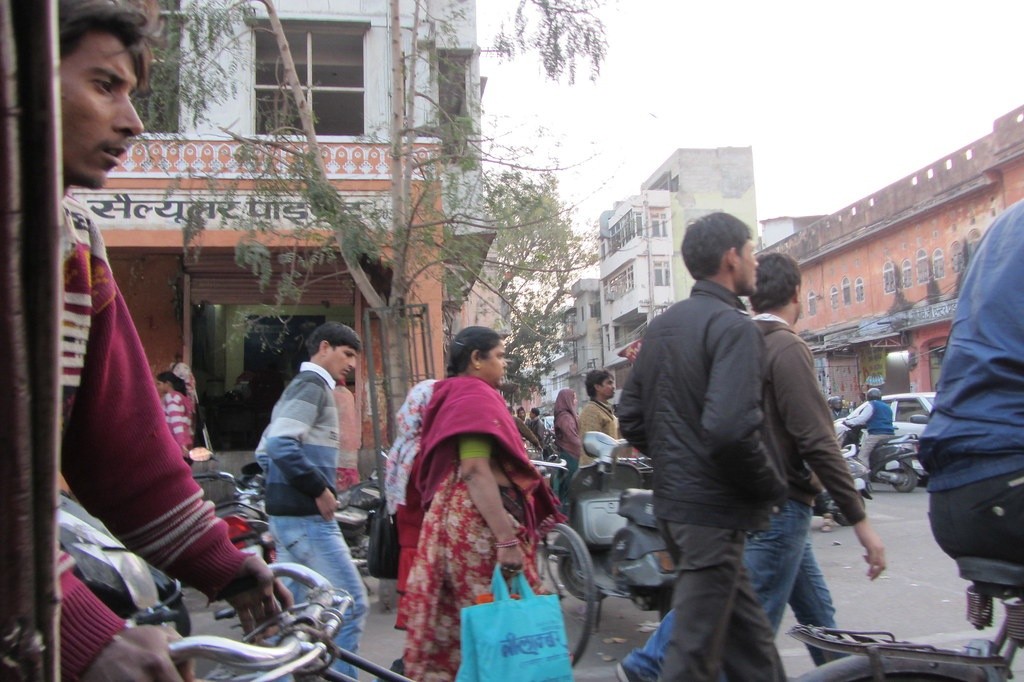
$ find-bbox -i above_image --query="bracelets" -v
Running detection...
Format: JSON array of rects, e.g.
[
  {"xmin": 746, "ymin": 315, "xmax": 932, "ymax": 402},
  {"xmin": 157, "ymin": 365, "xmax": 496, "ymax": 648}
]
[{"xmin": 494, "ymin": 539, "xmax": 518, "ymax": 548}]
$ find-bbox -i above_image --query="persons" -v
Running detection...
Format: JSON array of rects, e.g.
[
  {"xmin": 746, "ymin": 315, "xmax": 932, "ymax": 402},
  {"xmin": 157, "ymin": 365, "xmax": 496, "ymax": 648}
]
[
  {"xmin": 255, "ymin": 321, "xmax": 369, "ymax": 682},
  {"xmin": 828, "ymin": 388, "xmax": 896, "ymax": 490},
  {"xmin": 155, "ymin": 372, "xmax": 194, "ymax": 464},
  {"xmin": 918, "ymin": 198, "xmax": 1024, "ymax": 565},
  {"xmin": 59, "ymin": 0, "xmax": 300, "ymax": 682},
  {"xmin": 616, "ymin": 212, "xmax": 789, "ymax": 682},
  {"xmin": 815, "ymin": 493, "xmax": 833, "ymax": 533},
  {"xmin": 387, "ymin": 326, "xmax": 623, "ymax": 682},
  {"xmin": 617, "ymin": 252, "xmax": 888, "ymax": 682}
]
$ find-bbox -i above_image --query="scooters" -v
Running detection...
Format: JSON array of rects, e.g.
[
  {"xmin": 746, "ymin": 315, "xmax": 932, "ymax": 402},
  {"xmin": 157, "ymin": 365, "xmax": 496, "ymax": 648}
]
[
  {"xmin": 189, "ymin": 446, "xmax": 390, "ymax": 568},
  {"xmin": 557, "ymin": 430, "xmax": 680, "ymax": 624},
  {"xmin": 833, "ymin": 419, "xmax": 929, "ymax": 493},
  {"xmin": 58, "ymin": 493, "xmax": 191, "ymax": 639},
  {"xmin": 812, "ymin": 443, "xmax": 873, "ymax": 527}
]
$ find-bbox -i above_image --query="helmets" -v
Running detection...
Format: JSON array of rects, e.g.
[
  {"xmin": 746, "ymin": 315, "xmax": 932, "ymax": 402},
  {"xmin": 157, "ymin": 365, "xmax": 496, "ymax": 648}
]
[
  {"xmin": 867, "ymin": 388, "xmax": 881, "ymax": 400},
  {"xmin": 828, "ymin": 396, "xmax": 842, "ymax": 407}
]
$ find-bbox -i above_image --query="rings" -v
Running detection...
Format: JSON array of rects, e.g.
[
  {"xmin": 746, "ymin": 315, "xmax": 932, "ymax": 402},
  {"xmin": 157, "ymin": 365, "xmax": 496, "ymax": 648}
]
[
  {"xmin": 502, "ymin": 567, "xmax": 507, "ymax": 570},
  {"xmin": 510, "ymin": 570, "xmax": 515, "ymax": 572}
]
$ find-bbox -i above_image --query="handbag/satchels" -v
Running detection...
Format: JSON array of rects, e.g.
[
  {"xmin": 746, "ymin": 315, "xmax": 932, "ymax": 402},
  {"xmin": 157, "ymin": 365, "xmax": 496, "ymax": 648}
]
[{"xmin": 455, "ymin": 564, "xmax": 574, "ymax": 682}]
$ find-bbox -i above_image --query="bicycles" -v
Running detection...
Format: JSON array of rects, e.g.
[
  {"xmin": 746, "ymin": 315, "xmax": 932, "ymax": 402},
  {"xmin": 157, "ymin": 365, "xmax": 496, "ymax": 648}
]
[{"xmin": 167, "ymin": 446, "xmax": 1024, "ymax": 681}]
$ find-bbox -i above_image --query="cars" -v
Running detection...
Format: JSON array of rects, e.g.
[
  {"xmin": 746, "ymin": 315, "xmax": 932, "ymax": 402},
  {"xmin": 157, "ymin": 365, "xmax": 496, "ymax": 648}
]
[{"xmin": 848, "ymin": 392, "xmax": 938, "ymax": 438}]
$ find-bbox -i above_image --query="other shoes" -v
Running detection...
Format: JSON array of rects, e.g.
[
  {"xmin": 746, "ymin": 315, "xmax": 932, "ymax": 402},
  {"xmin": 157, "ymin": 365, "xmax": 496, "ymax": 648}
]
[{"xmin": 616, "ymin": 662, "xmax": 641, "ymax": 682}]
